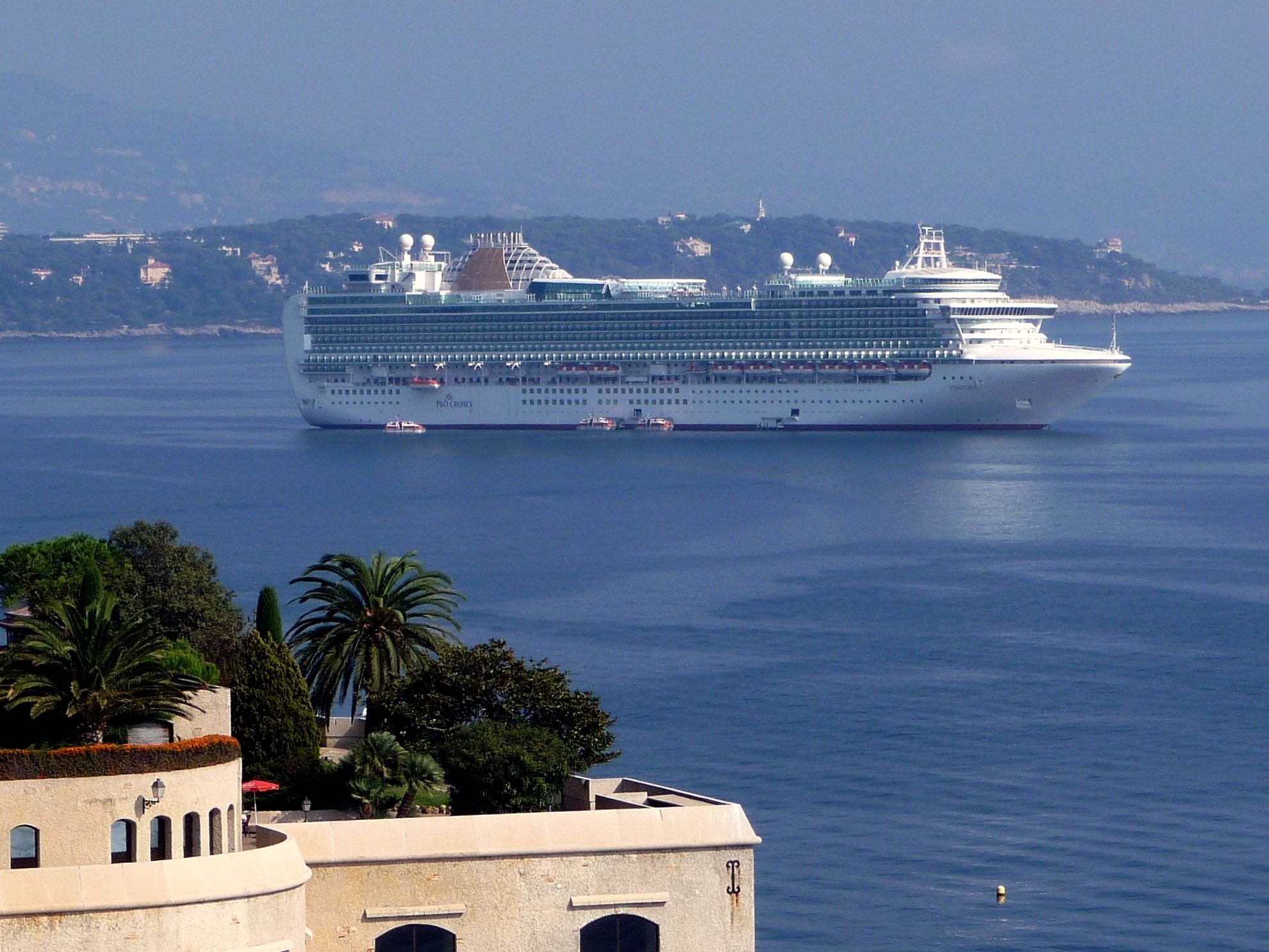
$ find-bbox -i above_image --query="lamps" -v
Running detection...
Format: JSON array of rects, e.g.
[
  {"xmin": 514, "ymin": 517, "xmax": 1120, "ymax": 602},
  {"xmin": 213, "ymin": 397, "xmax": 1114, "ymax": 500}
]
[
  {"xmin": 302, "ymin": 797, "xmax": 312, "ymax": 822},
  {"xmin": 143, "ymin": 779, "xmax": 166, "ymax": 814}
]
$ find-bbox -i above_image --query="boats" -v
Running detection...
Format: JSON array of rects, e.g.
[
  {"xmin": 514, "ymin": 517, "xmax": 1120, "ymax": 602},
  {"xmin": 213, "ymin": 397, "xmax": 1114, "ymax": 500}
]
[
  {"xmin": 587, "ymin": 364, "xmax": 619, "ymax": 376},
  {"xmin": 383, "ymin": 415, "xmax": 426, "ymax": 435},
  {"xmin": 555, "ymin": 365, "xmax": 586, "ymax": 378},
  {"xmin": 635, "ymin": 415, "xmax": 675, "ymax": 432},
  {"xmin": 820, "ymin": 362, "xmax": 853, "ymax": 375},
  {"xmin": 575, "ymin": 414, "xmax": 618, "ymax": 432},
  {"xmin": 855, "ymin": 361, "xmax": 891, "ymax": 376},
  {"xmin": 710, "ymin": 362, "xmax": 743, "ymax": 375},
  {"xmin": 783, "ymin": 362, "xmax": 816, "ymax": 375},
  {"xmin": 408, "ymin": 378, "xmax": 440, "ymax": 391},
  {"xmin": 744, "ymin": 363, "xmax": 776, "ymax": 375},
  {"xmin": 896, "ymin": 362, "xmax": 932, "ymax": 377},
  {"xmin": 685, "ymin": 363, "xmax": 707, "ymax": 373}
]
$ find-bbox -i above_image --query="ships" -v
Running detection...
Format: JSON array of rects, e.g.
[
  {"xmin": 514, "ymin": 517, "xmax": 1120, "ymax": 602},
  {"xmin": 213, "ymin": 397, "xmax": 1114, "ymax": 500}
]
[{"xmin": 280, "ymin": 223, "xmax": 1133, "ymax": 434}]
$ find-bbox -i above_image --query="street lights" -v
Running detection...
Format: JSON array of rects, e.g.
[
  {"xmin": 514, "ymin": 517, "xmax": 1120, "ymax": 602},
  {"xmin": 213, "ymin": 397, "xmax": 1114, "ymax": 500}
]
[{"xmin": 302, "ymin": 797, "xmax": 311, "ymax": 822}]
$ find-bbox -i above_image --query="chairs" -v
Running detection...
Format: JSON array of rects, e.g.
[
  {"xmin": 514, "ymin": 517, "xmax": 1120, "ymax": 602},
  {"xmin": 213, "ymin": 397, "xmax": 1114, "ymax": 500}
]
[{"xmin": 242, "ymin": 815, "xmax": 251, "ymax": 837}]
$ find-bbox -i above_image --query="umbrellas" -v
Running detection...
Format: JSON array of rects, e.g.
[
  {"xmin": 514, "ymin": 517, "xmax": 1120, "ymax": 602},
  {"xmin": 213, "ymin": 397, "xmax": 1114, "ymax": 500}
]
[{"xmin": 242, "ymin": 779, "xmax": 278, "ymax": 825}]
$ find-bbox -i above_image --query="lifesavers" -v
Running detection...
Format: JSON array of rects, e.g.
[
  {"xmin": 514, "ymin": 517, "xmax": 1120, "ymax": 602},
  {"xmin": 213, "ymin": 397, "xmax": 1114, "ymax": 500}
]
[
  {"xmin": 668, "ymin": 422, "xmax": 672, "ymax": 427},
  {"xmin": 664, "ymin": 422, "xmax": 668, "ymax": 426},
  {"xmin": 608, "ymin": 422, "xmax": 612, "ymax": 427},
  {"xmin": 611, "ymin": 422, "xmax": 615, "ymax": 426}
]
